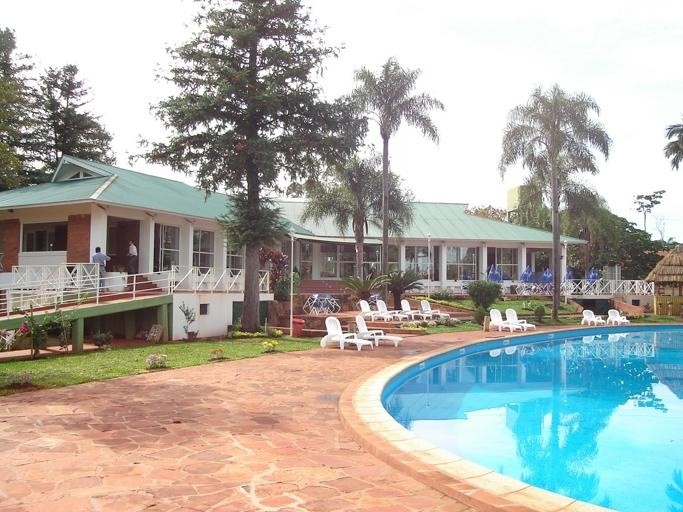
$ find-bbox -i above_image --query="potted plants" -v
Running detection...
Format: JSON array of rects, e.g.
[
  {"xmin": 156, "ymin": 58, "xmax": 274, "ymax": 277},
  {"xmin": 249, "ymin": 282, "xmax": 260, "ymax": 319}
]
[{"xmin": 177, "ymin": 301, "xmax": 199, "ymax": 343}]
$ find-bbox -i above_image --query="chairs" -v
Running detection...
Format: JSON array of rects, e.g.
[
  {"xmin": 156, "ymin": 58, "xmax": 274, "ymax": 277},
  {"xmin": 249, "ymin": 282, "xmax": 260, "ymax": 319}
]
[
  {"xmin": 580, "ymin": 309, "xmax": 630, "ymax": 327},
  {"xmin": 319, "ymin": 297, "xmax": 450, "ymax": 352},
  {"xmin": 488, "ymin": 308, "xmax": 535, "ymax": 332}
]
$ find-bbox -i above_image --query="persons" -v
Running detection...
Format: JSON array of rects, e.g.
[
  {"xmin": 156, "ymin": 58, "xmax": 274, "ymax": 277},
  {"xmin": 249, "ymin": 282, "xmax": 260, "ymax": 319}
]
[
  {"xmin": 128, "ymin": 240, "xmax": 138, "ymax": 274},
  {"xmin": 93, "ymin": 247, "xmax": 111, "ymax": 292}
]
